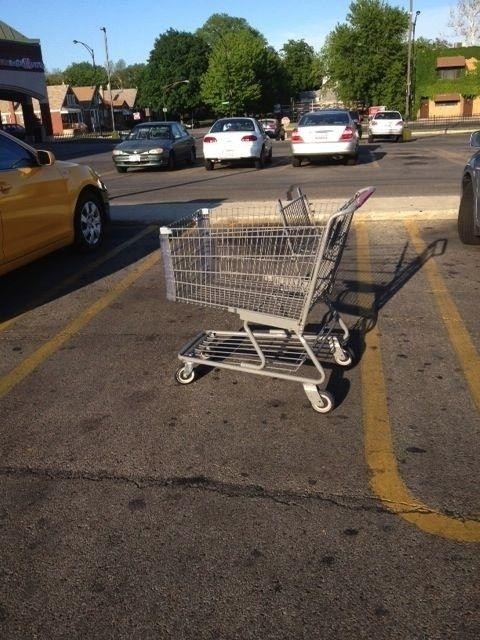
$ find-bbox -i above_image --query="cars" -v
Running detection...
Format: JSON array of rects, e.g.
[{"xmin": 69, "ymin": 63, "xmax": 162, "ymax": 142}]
[
  {"xmin": 185, "ymin": 118, "xmax": 201, "ymax": 129},
  {"xmin": 201, "ymin": 116, "xmax": 273, "ymax": 172},
  {"xmin": 290, "ymin": 108, "xmax": 360, "ymax": 167},
  {"xmin": 0, "ymin": 128, "xmax": 111, "ymax": 278},
  {"xmin": 111, "ymin": 119, "xmax": 197, "ymax": 172},
  {"xmin": 257, "ymin": 118, "xmax": 287, "ymax": 142},
  {"xmin": 2, "ymin": 123, "xmax": 26, "ymax": 141}
]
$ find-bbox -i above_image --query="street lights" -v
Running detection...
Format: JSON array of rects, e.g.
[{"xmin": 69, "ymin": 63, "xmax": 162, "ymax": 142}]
[
  {"xmin": 100, "ymin": 26, "xmax": 117, "ymax": 133},
  {"xmin": 73, "ymin": 39, "xmax": 104, "ymax": 137},
  {"xmin": 160, "ymin": 79, "xmax": 191, "ymax": 122},
  {"xmin": 412, "ymin": 10, "xmax": 421, "ymax": 118}
]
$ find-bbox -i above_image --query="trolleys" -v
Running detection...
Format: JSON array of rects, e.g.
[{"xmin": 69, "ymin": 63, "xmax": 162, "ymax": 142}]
[{"xmin": 157, "ymin": 181, "xmax": 378, "ymax": 415}]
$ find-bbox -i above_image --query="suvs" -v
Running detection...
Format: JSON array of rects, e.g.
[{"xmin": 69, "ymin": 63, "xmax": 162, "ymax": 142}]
[
  {"xmin": 367, "ymin": 109, "xmax": 405, "ymax": 144},
  {"xmin": 348, "ymin": 109, "xmax": 363, "ymax": 139},
  {"xmin": 454, "ymin": 126, "xmax": 480, "ymax": 246}
]
